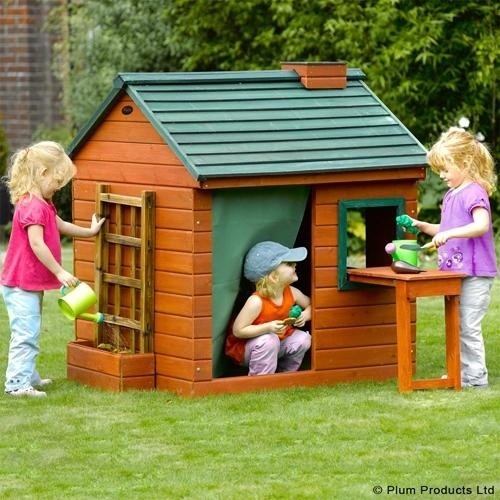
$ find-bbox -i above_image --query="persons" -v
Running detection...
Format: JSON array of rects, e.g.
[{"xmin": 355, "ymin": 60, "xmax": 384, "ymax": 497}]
[
  {"xmin": 0, "ymin": 140, "xmax": 108, "ymax": 399},
  {"xmin": 399, "ymin": 127, "xmax": 498, "ymax": 389},
  {"xmin": 225, "ymin": 241, "xmax": 314, "ymax": 378}
]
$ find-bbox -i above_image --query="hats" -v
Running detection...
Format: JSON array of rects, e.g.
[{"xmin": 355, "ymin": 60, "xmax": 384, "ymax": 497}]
[{"xmin": 244, "ymin": 241, "xmax": 308, "ymax": 282}]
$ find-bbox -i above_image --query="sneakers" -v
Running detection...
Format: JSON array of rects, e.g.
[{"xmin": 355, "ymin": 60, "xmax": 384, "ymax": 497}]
[
  {"xmin": 38, "ymin": 379, "xmax": 53, "ymax": 386},
  {"xmin": 442, "ymin": 372, "xmax": 488, "ymax": 387},
  {"xmin": 10, "ymin": 385, "xmax": 46, "ymax": 397}
]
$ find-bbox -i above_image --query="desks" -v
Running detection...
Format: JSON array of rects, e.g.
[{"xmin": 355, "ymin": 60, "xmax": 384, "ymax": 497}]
[{"xmin": 347, "ymin": 265, "xmax": 466, "ymax": 393}]
